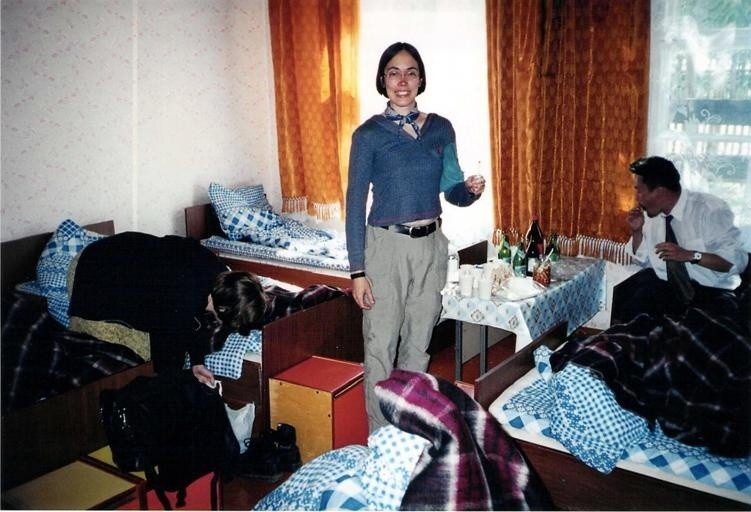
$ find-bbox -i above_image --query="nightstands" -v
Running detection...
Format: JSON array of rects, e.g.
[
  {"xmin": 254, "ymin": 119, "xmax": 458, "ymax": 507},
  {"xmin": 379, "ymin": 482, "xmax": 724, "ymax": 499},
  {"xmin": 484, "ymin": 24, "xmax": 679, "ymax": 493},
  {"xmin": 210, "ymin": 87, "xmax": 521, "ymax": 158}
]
[{"xmin": 269, "ymin": 355, "xmax": 367, "ymax": 466}]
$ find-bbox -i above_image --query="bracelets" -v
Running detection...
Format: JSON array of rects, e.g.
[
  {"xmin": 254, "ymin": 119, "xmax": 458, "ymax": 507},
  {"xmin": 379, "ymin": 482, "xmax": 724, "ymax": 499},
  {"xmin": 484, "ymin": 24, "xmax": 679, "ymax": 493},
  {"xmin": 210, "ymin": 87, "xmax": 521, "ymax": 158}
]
[{"xmin": 351, "ymin": 272, "xmax": 365, "ymax": 279}]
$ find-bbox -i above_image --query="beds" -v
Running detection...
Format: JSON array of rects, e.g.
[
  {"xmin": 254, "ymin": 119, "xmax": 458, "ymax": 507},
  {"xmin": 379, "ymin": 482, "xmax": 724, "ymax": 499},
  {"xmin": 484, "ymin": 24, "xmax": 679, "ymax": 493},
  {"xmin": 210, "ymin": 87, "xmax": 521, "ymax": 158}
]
[
  {"xmin": 184, "ymin": 195, "xmax": 488, "ymax": 363},
  {"xmin": 475, "ymin": 321, "xmax": 751, "ymax": 511},
  {"xmin": 0, "ymin": 220, "xmax": 364, "ymax": 428}
]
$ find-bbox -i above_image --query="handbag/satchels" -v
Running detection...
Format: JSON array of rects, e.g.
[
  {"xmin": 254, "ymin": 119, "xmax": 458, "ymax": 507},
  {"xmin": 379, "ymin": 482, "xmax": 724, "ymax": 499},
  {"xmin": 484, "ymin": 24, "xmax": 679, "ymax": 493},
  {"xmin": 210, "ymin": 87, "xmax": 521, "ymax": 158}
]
[
  {"xmin": 99, "ymin": 374, "xmax": 161, "ymax": 473},
  {"xmin": 113, "ymin": 369, "xmax": 241, "ymax": 493}
]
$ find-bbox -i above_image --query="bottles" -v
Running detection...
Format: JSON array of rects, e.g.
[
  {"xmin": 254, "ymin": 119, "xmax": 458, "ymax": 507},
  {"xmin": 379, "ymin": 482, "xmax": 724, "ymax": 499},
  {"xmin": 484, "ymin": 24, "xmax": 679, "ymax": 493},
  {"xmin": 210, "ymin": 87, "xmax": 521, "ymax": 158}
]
[
  {"xmin": 544, "ymin": 233, "xmax": 560, "ymax": 282},
  {"xmin": 525, "ymin": 214, "xmax": 544, "ymax": 264},
  {"xmin": 523, "ymin": 234, "xmax": 541, "ymax": 277},
  {"xmin": 445, "ymin": 238, "xmax": 460, "ymax": 282},
  {"xmin": 497, "ymin": 235, "xmax": 511, "ymax": 265},
  {"xmin": 512, "ymin": 236, "xmax": 527, "ymax": 278}
]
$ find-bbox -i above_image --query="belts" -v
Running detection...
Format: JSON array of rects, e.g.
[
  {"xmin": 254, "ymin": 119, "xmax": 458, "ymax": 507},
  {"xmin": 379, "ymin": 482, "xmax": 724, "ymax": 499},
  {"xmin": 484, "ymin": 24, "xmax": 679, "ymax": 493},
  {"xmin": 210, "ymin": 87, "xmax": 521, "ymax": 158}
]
[{"xmin": 380, "ymin": 217, "xmax": 442, "ymax": 239}]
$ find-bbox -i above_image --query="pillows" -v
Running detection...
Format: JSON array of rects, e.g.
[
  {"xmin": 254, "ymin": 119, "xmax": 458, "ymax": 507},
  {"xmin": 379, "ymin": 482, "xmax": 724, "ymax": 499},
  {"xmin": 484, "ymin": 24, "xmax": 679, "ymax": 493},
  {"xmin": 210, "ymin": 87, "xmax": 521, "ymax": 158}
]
[
  {"xmin": 206, "ymin": 180, "xmax": 286, "ymax": 242},
  {"xmin": 36, "ymin": 220, "xmax": 110, "ymax": 327}
]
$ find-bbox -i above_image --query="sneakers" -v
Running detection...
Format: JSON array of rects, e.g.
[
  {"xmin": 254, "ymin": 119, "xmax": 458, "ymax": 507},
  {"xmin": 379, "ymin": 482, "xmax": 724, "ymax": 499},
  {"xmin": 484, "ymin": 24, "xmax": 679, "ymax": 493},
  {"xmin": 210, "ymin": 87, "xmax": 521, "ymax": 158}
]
[
  {"xmin": 265, "ymin": 423, "xmax": 302, "ymax": 472},
  {"xmin": 228, "ymin": 429, "xmax": 282, "ymax": 483}
]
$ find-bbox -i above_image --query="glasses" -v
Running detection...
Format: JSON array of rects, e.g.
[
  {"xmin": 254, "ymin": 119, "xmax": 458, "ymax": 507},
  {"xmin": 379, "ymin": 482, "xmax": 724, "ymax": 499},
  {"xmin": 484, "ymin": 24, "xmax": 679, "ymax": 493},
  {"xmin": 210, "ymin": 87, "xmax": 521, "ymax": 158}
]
[
  {"xmin": 629, "ymin": 157, "xmax": 648, "ymax": 177},
  {"xmin": 384, "ymin": 69, "xmax": 419, "ymax": 80}
]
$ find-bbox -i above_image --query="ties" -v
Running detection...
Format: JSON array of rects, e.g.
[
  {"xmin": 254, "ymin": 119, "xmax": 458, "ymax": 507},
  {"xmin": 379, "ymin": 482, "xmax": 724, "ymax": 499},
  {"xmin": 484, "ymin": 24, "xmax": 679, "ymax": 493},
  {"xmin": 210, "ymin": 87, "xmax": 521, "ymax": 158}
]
[{"xmin": 665, "ymin": 214, "xmax": 695, "ymax": 304}]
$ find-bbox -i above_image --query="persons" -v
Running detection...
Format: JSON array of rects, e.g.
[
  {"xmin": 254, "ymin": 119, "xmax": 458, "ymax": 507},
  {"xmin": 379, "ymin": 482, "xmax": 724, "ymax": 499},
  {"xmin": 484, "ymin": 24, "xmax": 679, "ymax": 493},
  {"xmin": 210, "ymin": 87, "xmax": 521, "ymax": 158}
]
[
  {"xmin": 344, "ymin": 42, "xmax": 485, "ymax": 438},
  {"xmin": 65, "ymin": 231, "xmax": 268, "ymax": 386},
  {"xmin": 619, "ymin": 155, "xmax": 749, "ymax": 332}
]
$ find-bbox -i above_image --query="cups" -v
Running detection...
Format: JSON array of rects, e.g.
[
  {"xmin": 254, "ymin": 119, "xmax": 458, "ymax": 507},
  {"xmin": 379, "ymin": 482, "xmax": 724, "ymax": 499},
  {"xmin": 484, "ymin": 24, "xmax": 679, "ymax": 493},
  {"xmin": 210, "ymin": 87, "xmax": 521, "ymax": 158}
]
[
  {"xmin": 533, "ymin": 264, "xmax": 550, "ymax": 287},
  {"xmin": 459, "ymin": 274, "xmax": 474, "ymax": 297},
  {"xmin": 473, "ymin": 269, "xmax": 483, "ymax": 290},
  {"xmin": 478, "ymin": 280, "xmax": 494, "ymax": 300},
  {"xmin": 460, "ymin": 264, "xmax": 475, "ymax": 288}
]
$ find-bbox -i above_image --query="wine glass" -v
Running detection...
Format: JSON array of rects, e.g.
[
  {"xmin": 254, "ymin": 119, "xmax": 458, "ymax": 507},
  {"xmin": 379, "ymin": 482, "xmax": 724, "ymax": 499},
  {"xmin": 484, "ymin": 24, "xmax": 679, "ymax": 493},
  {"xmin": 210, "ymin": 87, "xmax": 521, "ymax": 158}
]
[{"xmin": 468, "ymin": 157, "xmax": 485, "ymax": 194}]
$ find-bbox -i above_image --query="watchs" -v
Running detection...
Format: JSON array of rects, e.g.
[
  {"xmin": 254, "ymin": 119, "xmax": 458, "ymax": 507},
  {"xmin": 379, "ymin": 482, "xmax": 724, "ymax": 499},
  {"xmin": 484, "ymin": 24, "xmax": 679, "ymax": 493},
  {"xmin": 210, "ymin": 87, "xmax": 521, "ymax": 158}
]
[{"xmin": 691, "ymin": 251, "xmax": 703, "ymax": 264}]
